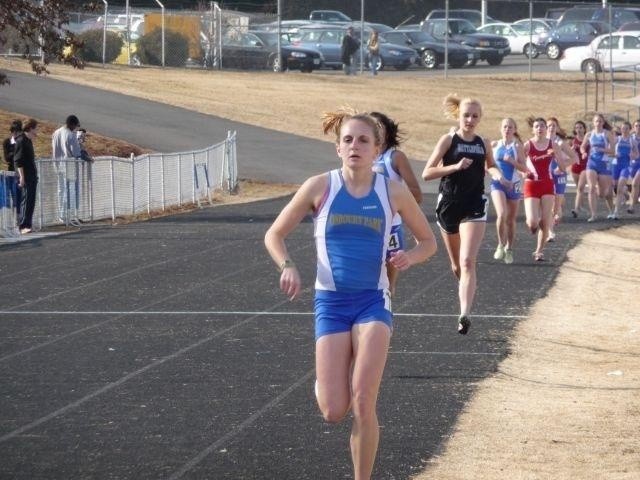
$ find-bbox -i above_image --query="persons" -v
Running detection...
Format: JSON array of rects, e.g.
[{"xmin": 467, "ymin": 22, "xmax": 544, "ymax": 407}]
[
  {"xmin": 488, "ymin": 117, "xmax": 529, "ymax": 266},
  {"xmin": 369, "ymin": 110, "xmax": 425, "ymax": 296},
  {"xmin": 366, "ymin": 29, "xmax": 380, "ymax": 76},
  {"xmin": 14, "ymin": 118, "xmax": 41, "ymax": 234},
  {"xmin": 263, "ymin": 106, "xmax": 438, "ymax": 480},
  {"xmin": 66, "ymin": 127, "xmax": 95, "ymax": 222},
  {"xmin": 524, "ymin": 112, "xmax": 640, "ymax": 262},
  {"xmin": 4, "ymin": 119, "xmax": 24, "ymax": 213},
  {"xmin": 424, "ymin": 91, "xmax": 515, "ymax": 334},
  {"xmin": 52, "ymin": 114, "xmax": 82, "ymax": 224},
  {"xmin": 341, "ymin": 26, "xmax": 362, "ymax": 75}
]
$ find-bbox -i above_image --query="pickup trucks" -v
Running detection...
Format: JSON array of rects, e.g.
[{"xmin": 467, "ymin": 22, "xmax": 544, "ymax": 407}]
[{"xmin": 397, "ymin": 18, "xmax": 511, "ymax": 68}]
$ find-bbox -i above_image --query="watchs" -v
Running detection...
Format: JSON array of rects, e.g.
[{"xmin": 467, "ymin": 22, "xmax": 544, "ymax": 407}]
[{"xmin": 276, "ymin": 259, "xmax": 297, "ymax": 271}]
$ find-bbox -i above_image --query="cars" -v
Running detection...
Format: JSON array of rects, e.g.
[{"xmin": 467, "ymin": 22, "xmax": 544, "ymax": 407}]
[
  {"xmin": 62, "ymin": 12, "xmax": 210, "ymax": 67},
  {"xmin": 380, "ymin": 29, "xmax": 481, "ymax": 71},
  {"xmin": 558, "ymin": 30, "xmax": 639, "ymax": 72},
  {"xmin": 476, "ymin": 22, "xmax": 543, "ymax": 59},
  {"xmin": 247, "ymin": 9, "xmax": 421, "ymax": 75},
  {"xmin": 217, "ymin": 30, "xmax": 324, "ymax": 73}
]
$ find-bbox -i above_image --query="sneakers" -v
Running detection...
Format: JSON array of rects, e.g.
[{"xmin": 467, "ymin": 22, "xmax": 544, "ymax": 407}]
[
  {"xmin": 494, "ymin": 244, "xmax": 513, "ymax": 264},
  {"xmin": 458, "ymin": 313, "xmax": 470, "ymax": 334},
  {"xmin": 546, "ymin": 232, "xmax": 555, "ymax": 242},
  {"xmin": 607, "ymin": 213, "xmax": 619, "ymax": 220},
  {"xmin": 572, "ymin": 209, "xmax": 578, "ymax": 218}
]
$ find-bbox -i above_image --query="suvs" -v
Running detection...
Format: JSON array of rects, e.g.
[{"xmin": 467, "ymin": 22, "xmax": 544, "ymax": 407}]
[
  {"xmin": 426, "ymin": 9, "xmax": 503, "ymax": 28},
  {"xmin": 508, "ymin": 5, "xmax": 640, "ymax": 60}
]
